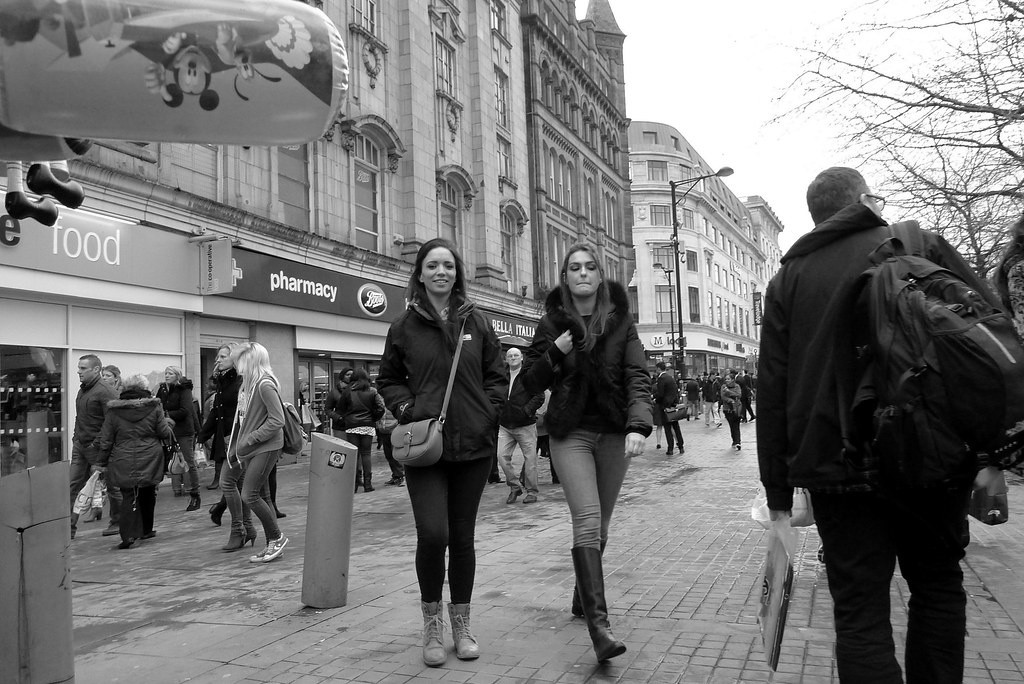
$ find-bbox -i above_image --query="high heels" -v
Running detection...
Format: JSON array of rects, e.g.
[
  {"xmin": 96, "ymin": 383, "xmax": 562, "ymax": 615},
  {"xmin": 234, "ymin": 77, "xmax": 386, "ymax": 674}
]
[{"xmin": 84, "ymin": 507, "xmax": 102, "ymax": 521}]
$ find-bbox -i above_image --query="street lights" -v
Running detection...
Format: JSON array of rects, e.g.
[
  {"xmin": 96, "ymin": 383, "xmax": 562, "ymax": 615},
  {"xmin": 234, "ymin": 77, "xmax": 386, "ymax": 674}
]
[
  {"xmin": 653, "ymin": 262, "xmax": 675, "ymax": 369},
  {"xmin": 669, "ymin": 166, "xmax": 734, "ymax": 380}
]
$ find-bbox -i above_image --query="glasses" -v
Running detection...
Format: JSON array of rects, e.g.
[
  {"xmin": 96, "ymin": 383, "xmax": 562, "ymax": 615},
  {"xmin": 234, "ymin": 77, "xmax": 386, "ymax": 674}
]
[{"xmin": 857, "ymin": 193, "xmax": 886, "ymax": 212}]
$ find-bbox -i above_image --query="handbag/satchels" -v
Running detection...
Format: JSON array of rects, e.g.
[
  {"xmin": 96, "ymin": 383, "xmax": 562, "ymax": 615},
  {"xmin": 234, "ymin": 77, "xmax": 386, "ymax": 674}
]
[
  {"xmin": 755, "ymin": 515, "xmax": 799, "ymax": 670},
  {"xmin": 162, "ymin": 425, "xmax": 190, "ymax": 475},
  {"xmin": 390, "ymin": 417, "xmax": 445, "ymax": 466},
  {"xmin": 301, "ymin": 402, "xmax": 322, "ymax": 428},
  {"xmin": 193, "ymin": 442, "xmax": 208, "ymax": 468},
  {"xmin": 732, "ymin": 397, "xmax": 742, "ymax": 417},
  {"xmin": 746, "ymin": 386, "xmax": 755, "ymax": 398},
  {"xmin": 751, "ymin": 484, "xmax": 816, "ymax": 529},
  {"xmin": 663, "ymin": 405, "xmax": 687, "ymax": 422},
  {"xmin": 72, "ymin": 470, "xmax": 102, "ymax": 515},
  {"xmin": 377, "ymin": 419, "xmax": 398, "ymax": 435}
]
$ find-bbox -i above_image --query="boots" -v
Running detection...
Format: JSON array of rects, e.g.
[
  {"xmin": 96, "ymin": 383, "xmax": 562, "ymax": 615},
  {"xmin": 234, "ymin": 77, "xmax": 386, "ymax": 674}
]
[
  {"xmin": 221, "ymin": 514, "xmax": 258, "ymax": 550},
  {"xmin": 571, "ymin": 541, "xmax": 628, "ymax": 663},
  {"xmin": 421, "ymin": 600, "xmax": 447, "ymax": 667},
  {"xmin": 447, "ymin": 603, "xmax": 481, "ymax": 662}
]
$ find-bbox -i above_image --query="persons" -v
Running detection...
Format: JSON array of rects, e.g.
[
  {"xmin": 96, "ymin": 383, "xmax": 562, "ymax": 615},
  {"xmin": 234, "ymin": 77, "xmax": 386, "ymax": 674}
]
[
  {"xmin": 325, "ymin": 344, "xmax": 757, "ymax": 504},
  {"xmin": 70, "ymin": 342, "xmax": 303, "ymax": 563},
  {"xmin": 757, "ymin": 166, "xmax": 1023, "ymax": 684},
  {"xmin": 515, "ymin": 244, "xmax": 654, "ymax": 664},
  {"xmin": 375, "ymin": 239, "xmax": 512, "ymax": 666}
]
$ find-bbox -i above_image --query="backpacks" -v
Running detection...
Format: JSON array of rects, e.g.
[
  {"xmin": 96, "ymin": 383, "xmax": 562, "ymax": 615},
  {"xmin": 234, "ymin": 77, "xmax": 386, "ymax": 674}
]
[
  {"xmin": 259, "ymin": 380, "xmax": 308, "ymax": 455},
  {"xmin": 832, "ymin": 218, "xmax": 1023, "ymax": 505}
]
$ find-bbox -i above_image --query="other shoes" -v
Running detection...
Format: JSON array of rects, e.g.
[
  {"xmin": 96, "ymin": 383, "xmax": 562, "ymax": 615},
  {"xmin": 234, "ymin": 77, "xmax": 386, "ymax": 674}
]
[
  {"xmin": 717, "ymin": 422, "xmax": 722, "ymax": 428},
  {"xmin": 749, "ymin": 416, "xmax": 756, "ymax": 421},
  {"xmin": 680, "ymin": 448, "xmax": 684, "ymax": 453},
  {"xmin": 186, "ymin": 460, "xmax": 286, "ymax": 526},
  {"xmin": 365, "ymin": 486, "xmax": 375, "ymax": 492},
  {"xmin": 732, "ymin": 441, "xmax": 741, "ymax": 451},
  {"xmin": 666, "ymin": 451, "xmax": 673, "ymax": 455},
  {"xmin": 740, "ymin": 419, "xmax": 747, "ymax": 423},
  {"xmin": 657, "ymin": 445, "xmax": 661, "ymax": 448},
  {"xmin": 817, "ymin": 545, "xmax": 825, "ymax": 564},
  {"xmin": 102, "ymin": 523, "xmax": 157, "ymax": 550},
  {"xmin": 384, "ymin": 475, "xmax": 404, "ymax": 486},
  {"xmin": 687, "ymin": 415, "xmax": 690, "ymax": 421}
]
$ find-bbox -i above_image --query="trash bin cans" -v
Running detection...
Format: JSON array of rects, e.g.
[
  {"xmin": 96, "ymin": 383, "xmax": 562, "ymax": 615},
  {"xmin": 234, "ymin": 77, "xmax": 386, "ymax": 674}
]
[
  {"xmin": 1, "ymin": 459, "xmax": 76, "ymax": 684},
  {"xmin": 27, "ymin": 408, "xmax": 48, "ymax": 466},
  {"xmin": 302, "ymin": 432, "xmax": 358, "ymax": 608}
]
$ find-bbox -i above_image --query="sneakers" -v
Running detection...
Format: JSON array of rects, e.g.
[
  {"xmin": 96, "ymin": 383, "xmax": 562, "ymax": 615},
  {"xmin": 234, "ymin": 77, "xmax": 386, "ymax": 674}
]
[{"xmin": 248, "ymin": 532, "xmax": 289, "ymax": 562}]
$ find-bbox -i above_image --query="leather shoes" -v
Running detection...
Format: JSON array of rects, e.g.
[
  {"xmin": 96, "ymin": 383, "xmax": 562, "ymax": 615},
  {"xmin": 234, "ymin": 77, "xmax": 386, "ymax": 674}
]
[{"xmin": 488, "ymin": 477, "xmax": 538, "ymax": 504}]
